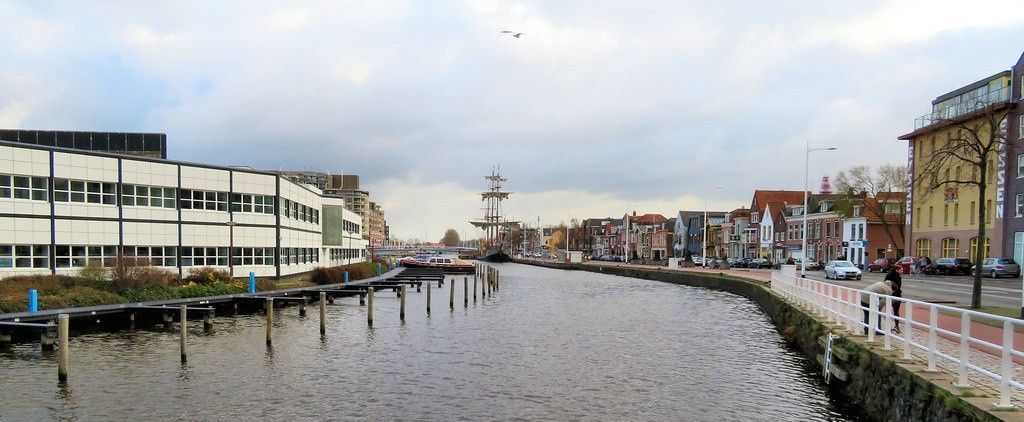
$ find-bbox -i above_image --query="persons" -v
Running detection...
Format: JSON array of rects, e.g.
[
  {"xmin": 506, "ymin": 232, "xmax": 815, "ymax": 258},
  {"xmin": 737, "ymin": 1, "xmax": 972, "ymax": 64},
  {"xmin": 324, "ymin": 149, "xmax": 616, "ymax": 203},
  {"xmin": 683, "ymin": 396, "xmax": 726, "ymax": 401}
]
[
  {"xmin": 861, "ymin": 281, "xmax": 898, "ymax": 335},
  {"xmin": 884, "ymin": 265, "xmax": 902, "ymax": 334},
  {"xmin": 913, "ymin": 256, "xmax": 932, "ymax": 271}
]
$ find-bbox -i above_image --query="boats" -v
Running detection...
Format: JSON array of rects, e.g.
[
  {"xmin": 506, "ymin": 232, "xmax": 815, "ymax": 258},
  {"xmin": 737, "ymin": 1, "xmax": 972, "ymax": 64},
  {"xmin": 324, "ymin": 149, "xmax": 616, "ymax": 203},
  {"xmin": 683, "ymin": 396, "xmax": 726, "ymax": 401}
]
[
  {"xmin": 457, "ymin": 249, "xmax": 475, "ymax": 260},
  {"xmin": 401, "ymin": 256, "xmax": 476, "ymax": 274}
]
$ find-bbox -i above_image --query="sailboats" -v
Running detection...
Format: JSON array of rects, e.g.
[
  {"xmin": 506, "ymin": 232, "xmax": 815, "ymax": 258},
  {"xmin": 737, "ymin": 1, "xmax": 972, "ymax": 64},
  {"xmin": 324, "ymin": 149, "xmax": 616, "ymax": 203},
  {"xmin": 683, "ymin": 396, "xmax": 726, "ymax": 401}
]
[{"xmin": 468, "ymin": 164, "xmax": 523, "ymax": 262}]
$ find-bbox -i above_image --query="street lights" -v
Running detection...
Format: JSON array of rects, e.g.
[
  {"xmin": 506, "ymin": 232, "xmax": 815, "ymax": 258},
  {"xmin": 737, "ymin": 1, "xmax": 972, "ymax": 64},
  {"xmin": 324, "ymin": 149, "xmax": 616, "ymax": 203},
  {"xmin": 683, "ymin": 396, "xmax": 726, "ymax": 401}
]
[
  {"xmin": 703, "ymin": 185, "xmax": 724, "ymax": 268},
  {"xmin": 566, "ymin": 212, "xmax": 576, "ymax": 260},
  {"xmin": 799, "ymin": 138, "xmax": 839, "ymax": 279},
  {"xmin": 626, "ymin": 211, "xmax": 636, "ymax": 263}
]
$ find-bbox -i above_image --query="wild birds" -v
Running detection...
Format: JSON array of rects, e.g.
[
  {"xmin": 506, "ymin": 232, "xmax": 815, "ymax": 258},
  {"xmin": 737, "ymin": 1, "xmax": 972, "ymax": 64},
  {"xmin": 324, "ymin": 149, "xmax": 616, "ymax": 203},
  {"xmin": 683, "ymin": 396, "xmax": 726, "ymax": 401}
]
[
  {"xmin": 501, "ymin": 31, "xmax": 511, "ymax": 33},
  {"xmin": 513, "ymin": 33, "xmax": 523, "ymax": 38}
]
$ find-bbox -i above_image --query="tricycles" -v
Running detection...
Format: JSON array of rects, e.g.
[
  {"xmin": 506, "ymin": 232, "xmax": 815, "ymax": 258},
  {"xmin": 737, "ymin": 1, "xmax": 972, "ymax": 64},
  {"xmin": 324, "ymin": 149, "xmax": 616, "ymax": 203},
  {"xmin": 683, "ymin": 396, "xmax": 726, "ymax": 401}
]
[{"xmin": 909, "ymin": 261, "xmax": 939, "ymax": 279}]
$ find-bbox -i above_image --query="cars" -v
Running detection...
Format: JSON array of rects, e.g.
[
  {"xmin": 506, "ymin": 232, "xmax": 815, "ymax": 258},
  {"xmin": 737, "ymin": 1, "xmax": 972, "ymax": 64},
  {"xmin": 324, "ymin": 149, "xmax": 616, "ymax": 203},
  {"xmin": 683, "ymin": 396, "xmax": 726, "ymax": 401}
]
[
  {"xmin": 795, "ymin": 257, "xmax": 820, "ymax": 271},
  {"xmin": 585, "ymin": 254, "xmax": 630, "ymax": 262},
  {"xmin": 930, "ymin": 258, "xmax": 973, "ymax": 276},
  {"xmin": 692, "ymin": 256, "xmax": 771, "ymax": 269},
  {"xmin": 823, "ymin": 260, "xmax": 859, "ymax": 281},
  {"xmin": 970, "ymin": 257, "xmax": 1021, "ymax": 279},
  {"xmin": 895, "ymin": 256, "xmax": 932, "ymax": 274},
  {"xmin": 775, "ymin": 258, "xmax": 796, "ymax": 269},
  {"xmin": 522, "ymin": 250, "xmax": 558, "ymax": 260},
  {"xmin": 868, "ymin": 257, "xmax": 900, "ymax": 273}
]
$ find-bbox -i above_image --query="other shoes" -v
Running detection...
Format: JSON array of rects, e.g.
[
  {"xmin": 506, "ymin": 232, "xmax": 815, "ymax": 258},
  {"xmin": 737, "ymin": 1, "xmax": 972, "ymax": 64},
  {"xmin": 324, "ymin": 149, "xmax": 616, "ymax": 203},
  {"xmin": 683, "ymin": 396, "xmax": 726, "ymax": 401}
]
[
  {"xmin": 893, "ymin": 327, "xmax": 901, "ymax": 335},
  {"xmin": 875, "ymin": 330, "xmax": 885, "ymax": 335},
  {"xmin": 865, "ymin": 331, "xmax": 876, "ymax": 335}
]
